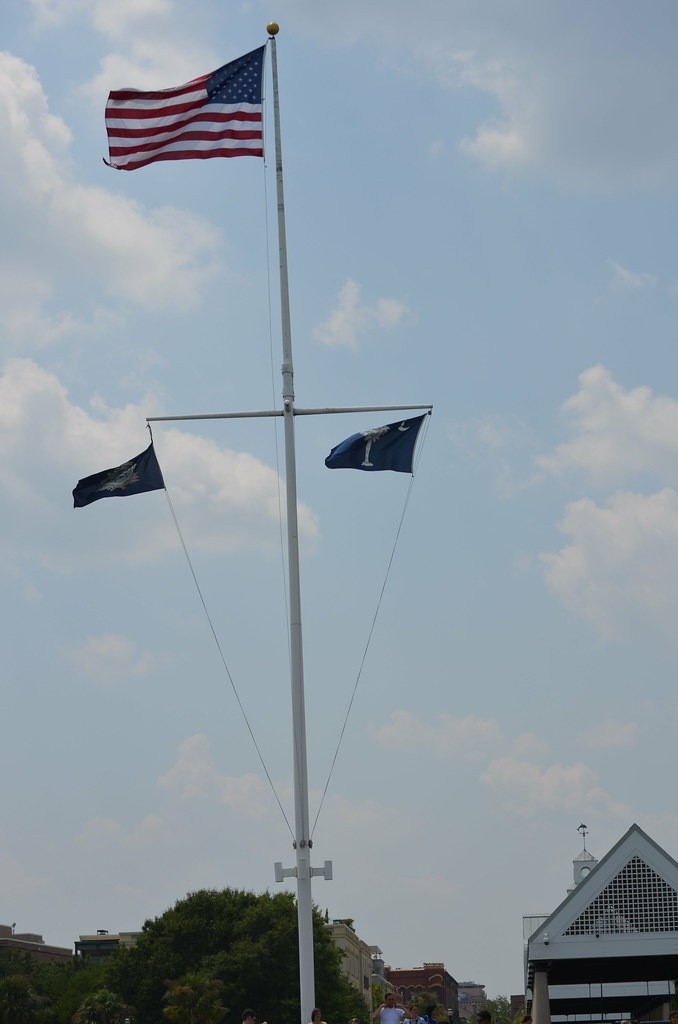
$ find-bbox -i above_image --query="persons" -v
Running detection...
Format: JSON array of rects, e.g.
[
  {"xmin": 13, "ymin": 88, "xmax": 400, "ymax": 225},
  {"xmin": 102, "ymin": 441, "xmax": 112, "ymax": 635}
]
[
  {"xmin": 669, "ymin": 1011, "xmax": 678, "ymax": 1024},
  {"xmin": 349, "ymin": 1018, "xmax": 360, "ymax": 1024},
  {"xmin": 475, "ymin": 1010, "xmax": 492, "ymax": 1024},
  {"xmin": 242, "ymin": 1009, "xmax": 258, "ymax": 1024},
  {"xmin": 309, "ymin": 1008, "xmax": 327, "ymax": 1024},
  {"xmin": 422, "ymin": 1005, "xmax": 440, "ymax": 1024},
  {"xmin": 403, "ymin": 1005, "xmax": 426, "ymax": 1024},
  {"xmin": 372, "ymin": 993, "xmax": 415, "ymax": 1024},
  {"xmin": 522, "ymin": 1015, "xmax": 532, "ymax": 1024}
]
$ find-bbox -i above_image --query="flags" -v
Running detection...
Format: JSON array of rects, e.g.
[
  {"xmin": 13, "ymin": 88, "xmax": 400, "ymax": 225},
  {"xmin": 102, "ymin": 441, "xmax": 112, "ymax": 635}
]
[
  {"xmin": 103, "ymin": 44, "xmax": 267, "ymax": 170},
  {"xmin": 326, "ymin": 412, "xmax": 428, "ymax": 473},
  {"xmin": 72, "ymin": 447, "xmax": 168, "ymax": 511}
]
